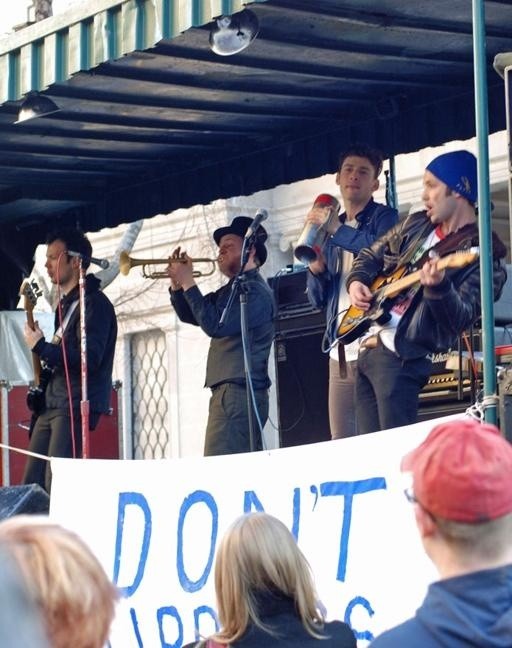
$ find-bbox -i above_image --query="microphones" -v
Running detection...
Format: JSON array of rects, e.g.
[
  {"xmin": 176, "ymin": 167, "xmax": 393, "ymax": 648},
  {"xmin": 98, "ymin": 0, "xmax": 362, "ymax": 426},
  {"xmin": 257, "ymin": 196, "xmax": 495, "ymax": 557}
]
[
  {"xmin": 244, "ymin": 207, "xmax": 268, "ymax": 241},
  {"xmin": 67, "ymin": 251, "xmax": 109, "ymax": 270}
]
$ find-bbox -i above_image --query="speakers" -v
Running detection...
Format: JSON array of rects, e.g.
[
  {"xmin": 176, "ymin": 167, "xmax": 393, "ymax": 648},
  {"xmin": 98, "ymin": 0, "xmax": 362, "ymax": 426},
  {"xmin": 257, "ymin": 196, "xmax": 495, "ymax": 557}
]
[
  {"xmin": 274, "ymin": 329, "xmax": 331, "ymax": 447},
  {"xmin": 0, "ymin": 483, "xmax": 51, "ymax": 520}
]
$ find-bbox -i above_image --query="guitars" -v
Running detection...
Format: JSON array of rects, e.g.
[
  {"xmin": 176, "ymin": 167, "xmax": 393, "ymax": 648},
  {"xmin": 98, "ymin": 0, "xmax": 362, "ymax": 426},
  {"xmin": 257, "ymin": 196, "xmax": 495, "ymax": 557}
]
[
  {"xmin": 20, "ymin": 279, "xmax": 52, "ymax": 439},
  {"xmin": 335, "ymin": 245, "xmax": 481, "ymax": 344}
]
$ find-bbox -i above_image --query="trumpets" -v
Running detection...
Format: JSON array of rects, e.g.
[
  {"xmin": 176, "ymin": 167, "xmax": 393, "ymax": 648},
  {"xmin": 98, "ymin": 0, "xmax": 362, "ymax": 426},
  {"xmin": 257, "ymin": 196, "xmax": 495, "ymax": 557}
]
[{"xmin": 119, "ymin": 252, "xmax": 224, "ymax": 279}]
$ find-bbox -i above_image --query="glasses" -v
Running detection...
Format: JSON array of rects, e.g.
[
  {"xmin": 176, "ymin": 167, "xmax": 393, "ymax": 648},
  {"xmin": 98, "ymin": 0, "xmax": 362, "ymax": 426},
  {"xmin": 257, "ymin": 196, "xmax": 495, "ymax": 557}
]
[{"xmin": 404, "ymin": 487, "xmax": 434, "ymax": 523}]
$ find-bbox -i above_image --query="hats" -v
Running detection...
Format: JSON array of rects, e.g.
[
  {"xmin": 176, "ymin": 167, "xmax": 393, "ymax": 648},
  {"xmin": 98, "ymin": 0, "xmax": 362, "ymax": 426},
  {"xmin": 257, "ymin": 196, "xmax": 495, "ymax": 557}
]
[
  {"xmin": 399, "ymin": 420, "xmax": 512, "ymax": 524},
  {"xmin": 426, "ymin": 151, "xmax": 478, "ymax": 202},
  {"xmin": 213, "ymin": 216, "xmax": 267, "ymax": 266}
]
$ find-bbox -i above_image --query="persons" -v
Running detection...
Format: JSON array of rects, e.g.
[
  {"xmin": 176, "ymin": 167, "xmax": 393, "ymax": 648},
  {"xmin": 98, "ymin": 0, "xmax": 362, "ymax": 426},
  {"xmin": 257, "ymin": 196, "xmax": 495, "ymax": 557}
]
[
  {"xmin": 304, "ymin": 134, "xmax": 397, "ymax": 440},
  {"xmin": 0, "ymin": 515, "xmax": 120, "ymax": 648},
  {"xmin": 180, "ymin": 512, "xmax": 357, "ymax": 648},
  {"xmin": 344, "ymin": 148, "xmax": 508, "ymax": 434},
  {"xmin": 366, "ymin": 419, "xmax": 512, "ymax": 648},
  {"xmin": 20, "ymin": 229, "xmax": 117, "ymax": 494},
  {"xmin": 166, "ymin": 216, "xmax": 278, "ymax": 456}
]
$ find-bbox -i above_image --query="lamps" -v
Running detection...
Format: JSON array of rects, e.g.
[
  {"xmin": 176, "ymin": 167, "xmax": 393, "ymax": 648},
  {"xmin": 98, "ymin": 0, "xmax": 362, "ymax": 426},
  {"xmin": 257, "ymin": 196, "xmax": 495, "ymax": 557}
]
[
  {"xmin": 14, "ymin": 90, "xmax": 60, "ymax": 127},
  {"xmin": 209, "ymin": 8, "xmax": 258, "ymax": 56}
]
